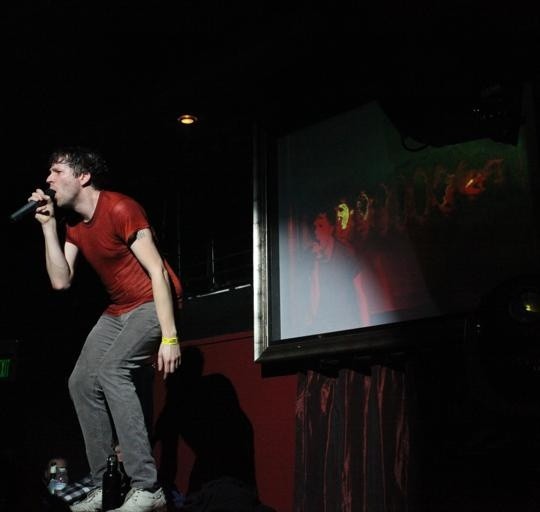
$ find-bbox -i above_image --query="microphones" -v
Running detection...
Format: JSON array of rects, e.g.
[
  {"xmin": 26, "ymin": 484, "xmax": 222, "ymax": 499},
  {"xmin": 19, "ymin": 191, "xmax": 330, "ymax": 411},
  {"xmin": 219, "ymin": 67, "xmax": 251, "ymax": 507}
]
[{"xmin": 10, "ymin": 189, "xmax": 55, "ymax": 220}]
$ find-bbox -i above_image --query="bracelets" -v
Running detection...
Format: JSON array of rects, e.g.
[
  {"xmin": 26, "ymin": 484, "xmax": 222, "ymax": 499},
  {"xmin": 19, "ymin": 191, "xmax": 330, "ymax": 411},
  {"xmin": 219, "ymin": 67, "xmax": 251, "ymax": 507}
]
[{"xmin": 160, "ymin": 335, "xmax": 181, "ymax": 346}]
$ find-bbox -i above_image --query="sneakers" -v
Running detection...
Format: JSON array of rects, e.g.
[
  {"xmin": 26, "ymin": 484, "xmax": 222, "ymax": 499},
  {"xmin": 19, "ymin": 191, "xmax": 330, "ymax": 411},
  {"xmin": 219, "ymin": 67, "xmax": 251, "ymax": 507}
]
[
  {"xmin": 106, "ymin": 484, "xmax": 167, "ymax": 512},
  {"xmin": 68, "ymin": 484, "xmax": 102, "ymax": 512}
]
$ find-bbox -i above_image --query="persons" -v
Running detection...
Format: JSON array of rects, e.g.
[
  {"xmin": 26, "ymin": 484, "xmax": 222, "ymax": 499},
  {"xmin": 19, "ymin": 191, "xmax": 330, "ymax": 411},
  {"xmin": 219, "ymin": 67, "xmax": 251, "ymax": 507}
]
[
  {"xmin": 25, "ymin": 133, "xmax": 186, "ymax": 510},
  {"xmin": 295, "ymin": 155, "xmax": 508, "ymax": 329}
]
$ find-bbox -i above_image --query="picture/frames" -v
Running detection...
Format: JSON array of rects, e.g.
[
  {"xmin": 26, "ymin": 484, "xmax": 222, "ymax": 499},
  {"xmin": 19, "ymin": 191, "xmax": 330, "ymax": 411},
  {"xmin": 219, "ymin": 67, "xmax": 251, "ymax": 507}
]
[{"xmin": 246, "ymin": 96, "xmax": 469, "ymax": 368}]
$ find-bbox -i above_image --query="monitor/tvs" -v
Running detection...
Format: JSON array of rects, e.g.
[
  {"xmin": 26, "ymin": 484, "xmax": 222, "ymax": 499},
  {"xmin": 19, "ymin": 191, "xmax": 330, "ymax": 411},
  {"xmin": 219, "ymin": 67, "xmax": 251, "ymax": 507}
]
[{"xmin": 251, "ymin": 80, "xmax": 540, "ymax": 364}]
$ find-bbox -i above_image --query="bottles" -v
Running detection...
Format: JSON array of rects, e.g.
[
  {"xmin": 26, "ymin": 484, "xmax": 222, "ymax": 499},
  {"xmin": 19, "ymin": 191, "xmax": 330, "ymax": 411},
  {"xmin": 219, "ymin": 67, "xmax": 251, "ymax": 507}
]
[
  {"xmin": 101, "ymin": 454, "xmax": 124, "ymax": 512},
  {"xmin": 48, "ymin": 461, "xmax": 69, "ymax": 496}
]
